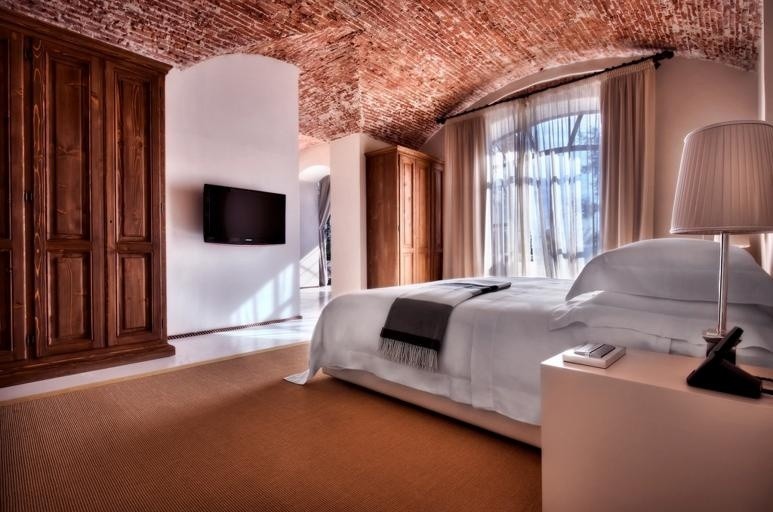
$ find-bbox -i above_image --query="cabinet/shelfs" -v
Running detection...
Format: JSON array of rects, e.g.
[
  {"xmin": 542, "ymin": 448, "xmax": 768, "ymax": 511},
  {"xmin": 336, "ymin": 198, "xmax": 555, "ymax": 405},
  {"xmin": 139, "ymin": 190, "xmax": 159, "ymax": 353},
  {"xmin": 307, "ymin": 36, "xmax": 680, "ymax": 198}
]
[
  {"xmin": 0, "ymin": 4, "xmax": 175, "ymax": 387},
  {"xmin": 365, "ymin": 144, "xmax": 443, "ymax": 288}
]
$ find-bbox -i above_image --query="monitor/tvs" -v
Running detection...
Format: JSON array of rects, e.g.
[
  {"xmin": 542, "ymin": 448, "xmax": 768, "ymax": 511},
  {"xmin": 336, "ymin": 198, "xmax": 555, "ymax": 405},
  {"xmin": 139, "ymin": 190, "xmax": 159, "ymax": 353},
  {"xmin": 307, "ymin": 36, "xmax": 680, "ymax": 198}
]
[{"xmin": 203, "ymin": 183, "xmax": 286, "ymax": 246}]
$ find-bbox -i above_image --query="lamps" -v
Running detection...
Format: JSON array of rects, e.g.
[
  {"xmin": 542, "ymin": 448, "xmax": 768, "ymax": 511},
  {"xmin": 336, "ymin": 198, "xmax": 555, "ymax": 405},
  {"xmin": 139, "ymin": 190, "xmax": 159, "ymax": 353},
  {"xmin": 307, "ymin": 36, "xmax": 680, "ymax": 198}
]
[{"xmin": 670, "ymin": 119, "xmax": 773, "ymax": 360}]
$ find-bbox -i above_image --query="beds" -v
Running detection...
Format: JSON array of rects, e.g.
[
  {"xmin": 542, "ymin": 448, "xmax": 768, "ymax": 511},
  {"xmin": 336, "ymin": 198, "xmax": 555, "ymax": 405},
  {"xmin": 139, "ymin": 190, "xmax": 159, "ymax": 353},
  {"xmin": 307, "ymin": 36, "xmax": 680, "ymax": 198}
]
[{"xmin": 283, "ymin": 276, "xmax": 773, "ymax": 451}]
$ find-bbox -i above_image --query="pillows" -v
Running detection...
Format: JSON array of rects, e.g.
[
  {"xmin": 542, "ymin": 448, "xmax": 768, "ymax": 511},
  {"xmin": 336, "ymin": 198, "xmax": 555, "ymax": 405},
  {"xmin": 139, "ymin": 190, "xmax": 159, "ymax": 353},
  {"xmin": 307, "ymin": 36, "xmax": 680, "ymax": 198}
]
[
  {"xmin": 564, "ymin": 235, "xmax": 773, "ymax": 307},
  {"xmin": 549, "ymin": 290, "xmax": 773, "ymax": 354}
]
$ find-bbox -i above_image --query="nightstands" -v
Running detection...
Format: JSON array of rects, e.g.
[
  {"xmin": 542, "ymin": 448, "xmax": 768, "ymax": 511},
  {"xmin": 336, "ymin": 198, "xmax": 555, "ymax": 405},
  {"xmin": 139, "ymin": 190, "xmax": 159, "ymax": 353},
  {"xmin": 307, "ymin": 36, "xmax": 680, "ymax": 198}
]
[{"xmin": 540, "ymin": 345, "xmax": 773, "ymax": 512}]
